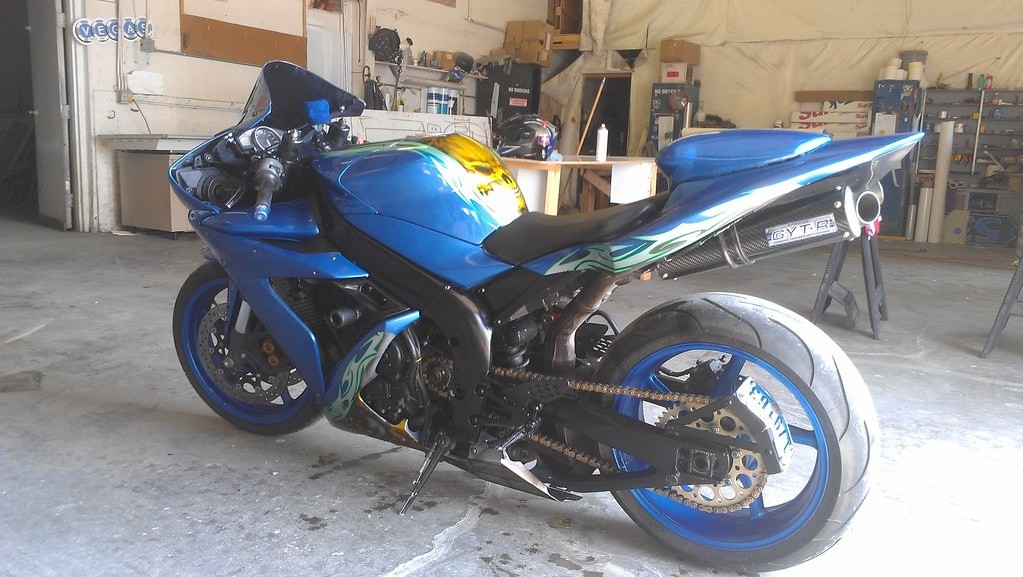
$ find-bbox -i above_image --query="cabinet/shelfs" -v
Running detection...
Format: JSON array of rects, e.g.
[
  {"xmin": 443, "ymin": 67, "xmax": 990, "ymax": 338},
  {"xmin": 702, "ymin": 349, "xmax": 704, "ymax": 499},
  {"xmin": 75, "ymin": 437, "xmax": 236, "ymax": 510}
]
[{"xmin": 788, "ymin": 87, "xmax": 1023, "ymax": 250}]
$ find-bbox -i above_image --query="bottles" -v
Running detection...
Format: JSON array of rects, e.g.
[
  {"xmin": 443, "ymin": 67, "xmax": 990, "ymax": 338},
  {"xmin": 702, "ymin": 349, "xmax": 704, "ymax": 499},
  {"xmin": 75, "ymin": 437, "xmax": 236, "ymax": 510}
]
[
  {"xmin": 978, "ymin": 73, "xmax": 984, "ymax": 91},
  {"xmin": 596, "ymin": 123, "xmax": 608, "ymax": 162},
  {"xmin": 985, "ymin": 73, "xmax": 993, "ymax": 90},
  {"xmin": 968, "ymin": 73, "xmax": 972, "ymax": 90},
  {"xmin": 993, "ymin": 93, "xmax": 998, "ymax": 105}
]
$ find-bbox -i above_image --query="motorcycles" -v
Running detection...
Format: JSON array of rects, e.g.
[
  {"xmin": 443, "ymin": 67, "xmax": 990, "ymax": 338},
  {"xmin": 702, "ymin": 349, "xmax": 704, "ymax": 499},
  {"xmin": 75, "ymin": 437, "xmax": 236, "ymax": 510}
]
[{"xmin": 167, "ymin": 58, "xmax": 925, "ymax": 574}]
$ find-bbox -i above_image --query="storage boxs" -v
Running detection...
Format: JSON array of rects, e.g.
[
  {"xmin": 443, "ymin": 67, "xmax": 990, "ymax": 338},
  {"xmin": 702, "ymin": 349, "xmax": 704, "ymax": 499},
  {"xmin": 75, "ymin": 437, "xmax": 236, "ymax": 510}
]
[
  {"xmin": 502, "ymin": 20, "xmax": 556, "ymax": 68},
  {"xmin": 659, "ymin": 39, "xmax": 702, "ymax": 66}
]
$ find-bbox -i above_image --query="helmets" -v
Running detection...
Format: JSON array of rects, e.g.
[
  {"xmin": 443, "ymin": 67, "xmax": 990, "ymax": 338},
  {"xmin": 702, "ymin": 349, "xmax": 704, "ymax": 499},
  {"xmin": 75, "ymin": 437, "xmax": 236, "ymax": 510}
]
[{"xmin": 494, "ymin": 113, "xmax": 559, "ymax": 162}]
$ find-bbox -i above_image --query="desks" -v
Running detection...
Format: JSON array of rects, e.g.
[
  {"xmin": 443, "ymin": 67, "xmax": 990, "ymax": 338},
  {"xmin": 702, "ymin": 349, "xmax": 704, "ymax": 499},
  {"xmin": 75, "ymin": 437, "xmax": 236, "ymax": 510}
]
[{"xmin": 502, "ymin": 153, "xmax": 661, "ymax": 216}]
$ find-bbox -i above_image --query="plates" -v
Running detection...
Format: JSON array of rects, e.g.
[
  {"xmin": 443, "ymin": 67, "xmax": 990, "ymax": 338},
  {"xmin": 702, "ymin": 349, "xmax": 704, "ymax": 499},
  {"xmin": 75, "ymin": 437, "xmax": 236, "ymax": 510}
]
[
  {"xmin": 878, "ymin": 58, "xmax": 907, "ymax": 80},
  {"xmin": 908, "ymin": 61, "xmax": 923, "ymax": 81}
]
